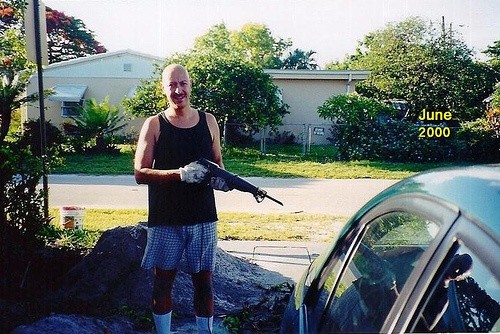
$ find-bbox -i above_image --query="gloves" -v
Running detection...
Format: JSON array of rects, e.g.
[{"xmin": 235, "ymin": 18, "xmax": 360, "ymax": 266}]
[
  {"xmin": 179, "ymin": 161, "xmax": 208, "ymax": 183},
  {"xmin": 210, "ymin": 177, "xmax": 229, "ymax": 191}
]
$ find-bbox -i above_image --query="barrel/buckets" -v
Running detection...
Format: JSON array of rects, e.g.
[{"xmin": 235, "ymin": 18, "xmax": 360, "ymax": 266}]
[{"xmin": 60, "ymin": 206, "xmax": 85, "ymax": 232}]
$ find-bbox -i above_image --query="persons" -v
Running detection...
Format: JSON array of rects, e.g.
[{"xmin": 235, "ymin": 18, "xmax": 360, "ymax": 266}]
[
  {"xmin": 134, "ymin": 62, "xmax": 229, "ymax": 334},
  {"xmin": 328, "ymin": 241, "xmax": 473, "ymax": 334}
]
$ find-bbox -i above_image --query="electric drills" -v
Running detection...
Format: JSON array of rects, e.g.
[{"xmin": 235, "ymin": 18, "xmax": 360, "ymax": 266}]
[{"xmin": 198, "ymin": 157, "xmax": 284, "ymax": 205}]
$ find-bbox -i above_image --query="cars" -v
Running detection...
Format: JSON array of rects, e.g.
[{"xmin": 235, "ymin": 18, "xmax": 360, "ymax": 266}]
[{"xmin": 279, "ymin": 163, "xmax": 500, "ymax": 334}]
